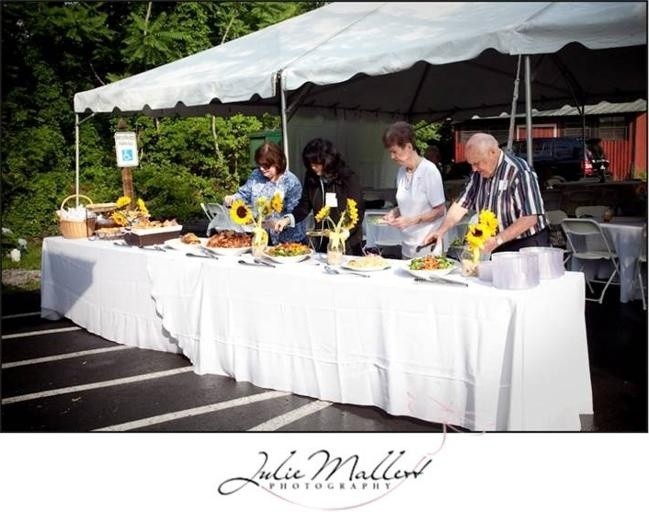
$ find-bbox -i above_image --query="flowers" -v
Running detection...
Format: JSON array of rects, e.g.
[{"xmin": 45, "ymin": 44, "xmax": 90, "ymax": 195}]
[
  {"xmin": 229, "ymin": 192, "xmax": 284, "ymax": 245},
  {"xmin": 315, "ymin": 198, "xmax": 359, "ymax": 249},
  {"xmin": 112, "ymin": 196, "xmax": 148, "ymax": 227},
  {"xmin": 463, "ymin": 209, "xmax": 498, "ymax": 262}
]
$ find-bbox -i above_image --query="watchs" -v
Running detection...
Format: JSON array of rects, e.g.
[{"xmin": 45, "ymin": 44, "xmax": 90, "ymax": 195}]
[{"xmin": 495, "ymin": 236, "xmax": 503, "ymax": 245}]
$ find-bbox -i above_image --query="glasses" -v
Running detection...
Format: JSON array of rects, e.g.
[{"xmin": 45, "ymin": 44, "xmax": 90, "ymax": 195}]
[{"xmin": 255, "ymin": 161, "xmax": 274, "ymax": 169}]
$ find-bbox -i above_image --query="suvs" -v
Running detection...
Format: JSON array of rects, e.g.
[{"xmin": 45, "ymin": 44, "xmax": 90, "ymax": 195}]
[{"xmin": 451, "ymin": 137, "xmax": 614, "ymax": 192}]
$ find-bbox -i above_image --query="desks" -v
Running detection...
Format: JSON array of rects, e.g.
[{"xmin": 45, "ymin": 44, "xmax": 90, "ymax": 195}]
[{"xmin": 40, "ymin": 237, "xmax": 595, "ymax": 433}]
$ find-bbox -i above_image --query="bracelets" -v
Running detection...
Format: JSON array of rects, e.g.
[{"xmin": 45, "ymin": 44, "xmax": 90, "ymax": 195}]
[{"xmin": 416, "ymin": 215, "xmax": 423, "ymax": 224}]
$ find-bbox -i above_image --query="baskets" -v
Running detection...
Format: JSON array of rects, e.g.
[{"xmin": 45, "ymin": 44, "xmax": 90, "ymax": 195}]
[{"xmin": 59, "ymin": 195, "xmax": 96, "ymax": 238}]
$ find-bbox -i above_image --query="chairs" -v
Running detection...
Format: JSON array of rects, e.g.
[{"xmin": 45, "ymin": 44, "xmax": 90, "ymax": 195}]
[
  {"xmin": 364, "ymin": 212, "xmax": 389, "ymax": 256},
  {"xmin": 545, "ymin": 206, "xmax": 648, "ymax": 311}
]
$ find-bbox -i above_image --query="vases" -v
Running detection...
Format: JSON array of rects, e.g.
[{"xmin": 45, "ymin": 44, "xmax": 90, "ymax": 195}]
[
  {"xmin": 251, "ymin": 230, "xmax": 266, "ymax": 256},
  {"xmin": 461, "ymin": 244, "xmax": 480, "ymax": 277},
  {"xmin": 327, "ymin": 237, "xmax": 342, "ymax": 265}
]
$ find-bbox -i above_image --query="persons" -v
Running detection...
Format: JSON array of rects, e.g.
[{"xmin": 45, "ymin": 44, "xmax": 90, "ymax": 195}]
[
  {"xmin": 274, "ymin": 138, "xmax": 365, "ymax": 257},
  {"xmin": 424, "ymin": 133, "xmax": 548, "ymax": 263},
  {"xmin": 229, "ymin": 143, "xmax": 308, "ymax": 242},
  {"xmin": 383, "ymin": 121, "xmax": 448, "ymax": 259}
]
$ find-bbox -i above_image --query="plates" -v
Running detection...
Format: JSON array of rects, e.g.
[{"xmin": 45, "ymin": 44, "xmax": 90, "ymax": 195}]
[
  {"xmin": 110, "ymin": 232, "xmax": 463, "ymax": 290},
  {"xmin": 477, "ymin": 245, "xmax": 571, "ymax": 288}
]
